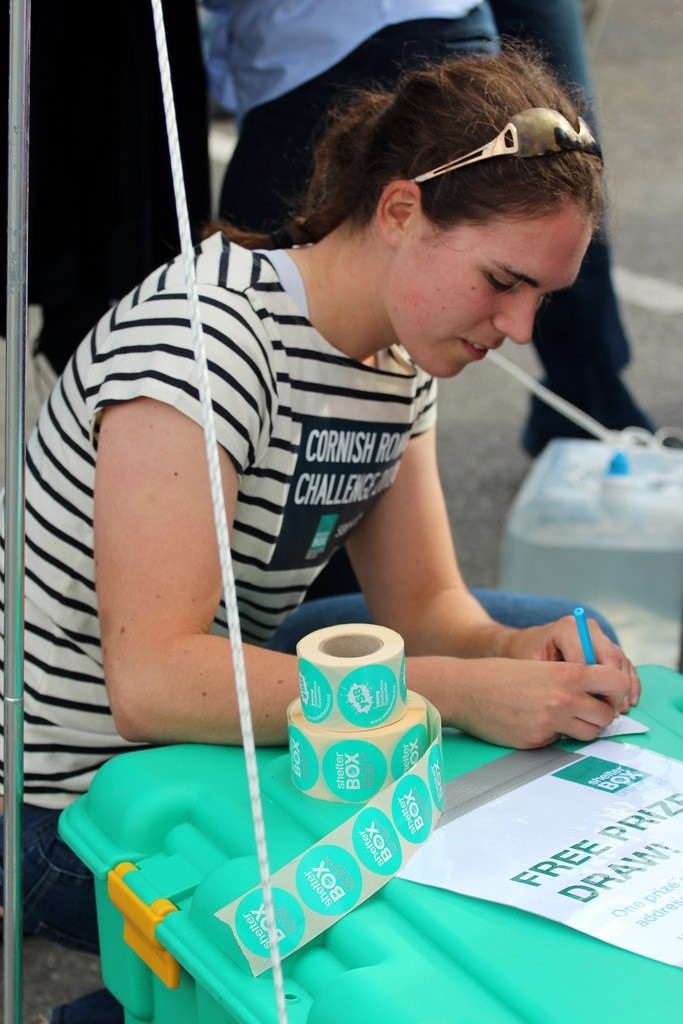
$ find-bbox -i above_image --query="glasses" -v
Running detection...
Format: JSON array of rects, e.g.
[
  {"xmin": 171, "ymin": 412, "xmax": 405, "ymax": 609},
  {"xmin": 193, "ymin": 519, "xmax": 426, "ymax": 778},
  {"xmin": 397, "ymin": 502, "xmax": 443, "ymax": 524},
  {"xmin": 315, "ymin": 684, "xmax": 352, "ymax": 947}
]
[{"xmin": 382, "ymin": 108, "xmax": 604, "ymax": 192}]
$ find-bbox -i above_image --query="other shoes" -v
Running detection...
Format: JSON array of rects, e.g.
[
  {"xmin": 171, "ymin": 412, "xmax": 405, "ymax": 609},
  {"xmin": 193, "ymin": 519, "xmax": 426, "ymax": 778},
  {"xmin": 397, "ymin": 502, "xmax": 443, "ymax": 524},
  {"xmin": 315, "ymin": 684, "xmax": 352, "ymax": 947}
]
[{"xmin": 522, "ymin": 373, "xmax": 683, "ymax": 459}]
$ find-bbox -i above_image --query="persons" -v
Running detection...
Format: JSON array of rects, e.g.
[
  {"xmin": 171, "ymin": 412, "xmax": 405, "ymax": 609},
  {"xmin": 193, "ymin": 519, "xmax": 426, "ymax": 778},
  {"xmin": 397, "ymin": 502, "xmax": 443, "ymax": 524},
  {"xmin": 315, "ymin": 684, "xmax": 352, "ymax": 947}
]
[
  {"xmin": 0, "ymin": 49, "xmax": 643, "ymax": 1024},
  {"xmin": 0, "ymin": 0, "xmax": 682, "ymax": 613}
]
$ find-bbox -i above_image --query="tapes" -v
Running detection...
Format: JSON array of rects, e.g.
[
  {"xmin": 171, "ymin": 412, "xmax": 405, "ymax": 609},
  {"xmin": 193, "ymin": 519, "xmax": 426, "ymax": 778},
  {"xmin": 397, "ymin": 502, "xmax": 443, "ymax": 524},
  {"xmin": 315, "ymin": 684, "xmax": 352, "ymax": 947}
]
[{"xmin": 212, "ymin": 623, "xmax": 446, "ymax": 981}]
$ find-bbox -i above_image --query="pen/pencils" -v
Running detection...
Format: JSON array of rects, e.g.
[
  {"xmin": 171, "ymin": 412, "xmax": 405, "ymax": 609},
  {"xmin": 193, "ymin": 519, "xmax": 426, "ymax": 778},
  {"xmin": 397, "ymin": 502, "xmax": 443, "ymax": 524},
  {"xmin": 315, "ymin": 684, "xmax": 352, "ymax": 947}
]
[{"xmin": 572, "ymin": 608, "xmax": 604, "ymax": 701}]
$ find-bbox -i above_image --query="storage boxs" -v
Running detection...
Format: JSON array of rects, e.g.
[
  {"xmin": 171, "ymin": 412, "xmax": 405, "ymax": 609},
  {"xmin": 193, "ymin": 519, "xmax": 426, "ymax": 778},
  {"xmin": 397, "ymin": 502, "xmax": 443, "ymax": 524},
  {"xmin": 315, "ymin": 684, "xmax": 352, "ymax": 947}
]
[{"xmin": 57, "ymin": 664, "xmax": 683, "ymax": 1024}]
